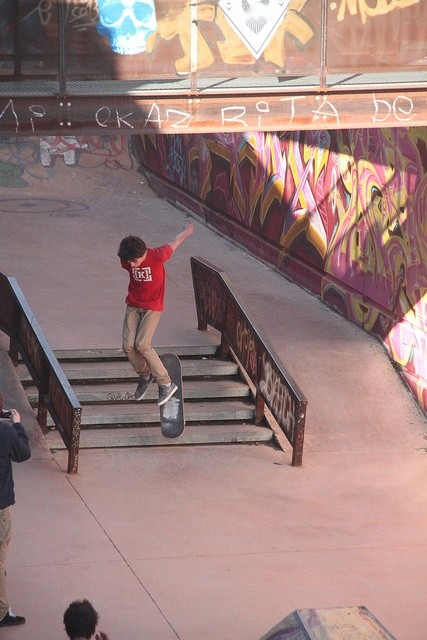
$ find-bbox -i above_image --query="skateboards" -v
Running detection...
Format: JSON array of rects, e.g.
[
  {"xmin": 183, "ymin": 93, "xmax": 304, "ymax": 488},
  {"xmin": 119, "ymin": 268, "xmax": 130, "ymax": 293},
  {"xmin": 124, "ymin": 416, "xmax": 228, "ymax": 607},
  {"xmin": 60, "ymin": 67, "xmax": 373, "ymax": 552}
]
[{"xmin": 159, "ymin": 353, "xmax": 185, "ymax": 439}]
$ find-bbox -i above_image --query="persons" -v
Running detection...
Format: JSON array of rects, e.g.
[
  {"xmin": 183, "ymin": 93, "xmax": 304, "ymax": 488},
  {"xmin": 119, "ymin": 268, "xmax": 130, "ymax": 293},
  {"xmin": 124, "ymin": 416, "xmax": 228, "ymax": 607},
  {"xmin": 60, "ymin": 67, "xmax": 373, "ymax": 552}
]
[
  {"xmin": 63, "ymin": 598, "xmax": 109, "ymax": 640},
  {"xmin": 0, "ymin": 393, "xmax": 30, "ymax": 627},
  {"xmin": 118, "ymin": 218, "xmax": 196, "ymax": 407}
]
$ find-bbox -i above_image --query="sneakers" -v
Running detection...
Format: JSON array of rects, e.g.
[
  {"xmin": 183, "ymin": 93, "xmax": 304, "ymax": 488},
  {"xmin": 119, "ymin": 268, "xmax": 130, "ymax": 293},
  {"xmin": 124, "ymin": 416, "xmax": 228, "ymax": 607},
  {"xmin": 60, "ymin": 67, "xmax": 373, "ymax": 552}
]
[
  {"xmin": 135, "ymin": 374, "xmax": 155, "ymax": 402},
  {"xmin": 1, "ymin": 613, "xmax": 26, "ymax": 627},
  {"xmin": 157, "ymin": 382, "xmax": 179, "ymax": 408}
]
